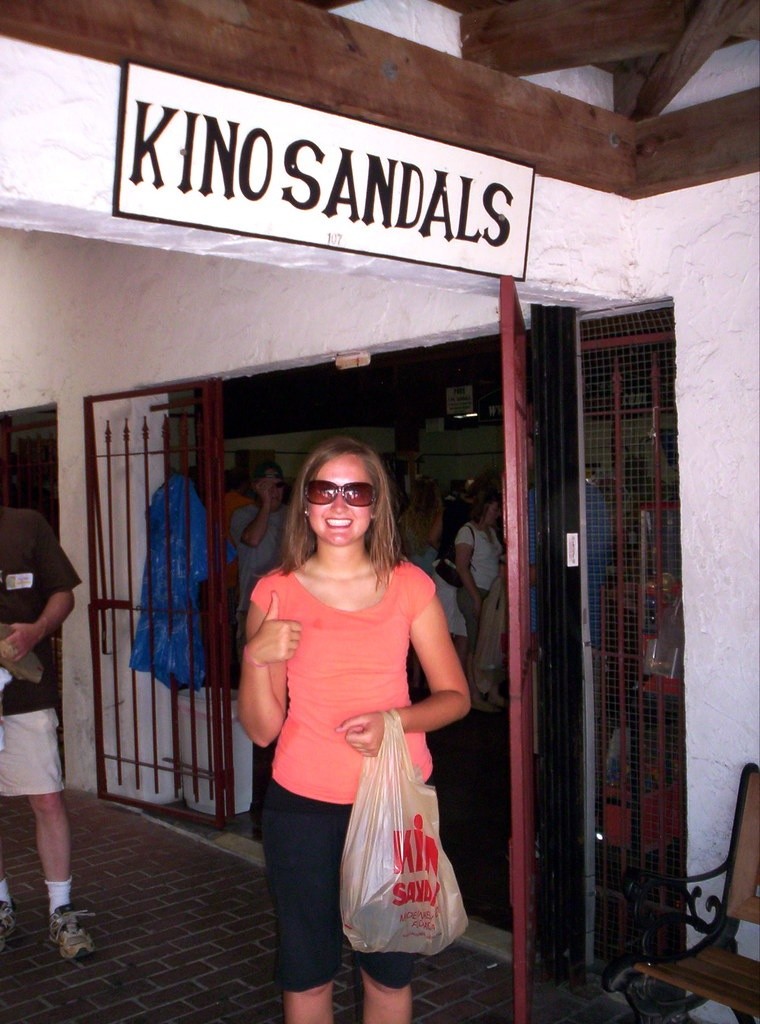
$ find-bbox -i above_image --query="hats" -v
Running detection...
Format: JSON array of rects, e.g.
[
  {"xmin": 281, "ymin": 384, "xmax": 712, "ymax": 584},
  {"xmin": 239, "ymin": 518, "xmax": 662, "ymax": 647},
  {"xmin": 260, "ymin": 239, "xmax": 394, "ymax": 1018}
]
[{"xmin": 254, "ymin": 460, "xmax": 285, "ymax": 480}]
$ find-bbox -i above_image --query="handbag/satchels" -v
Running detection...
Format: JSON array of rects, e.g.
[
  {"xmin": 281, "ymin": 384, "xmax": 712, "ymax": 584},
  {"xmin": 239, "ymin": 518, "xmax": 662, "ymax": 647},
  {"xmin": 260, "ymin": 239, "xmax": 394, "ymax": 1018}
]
[
  {"xmin": 433, "ymin": 524, "xmax": 476, "ymax": 587},
  {"xmin": 338, "ymin": 708, "xmax": 470, "ymax": 958}
]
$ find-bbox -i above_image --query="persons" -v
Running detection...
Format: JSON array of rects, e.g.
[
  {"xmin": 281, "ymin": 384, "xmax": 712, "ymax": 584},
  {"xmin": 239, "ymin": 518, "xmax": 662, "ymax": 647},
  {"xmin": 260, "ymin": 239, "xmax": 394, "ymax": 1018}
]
[
  {"xmin": 168, "ymin": 460, "xmax": 683, "ymax": 714},
  {"xmin": 1, "ymin": 493, "xmax": 99, "ymax": 964},
  {"xmin": 238, "ymin": 432, "xmax": 472, "ymax": 1024}
]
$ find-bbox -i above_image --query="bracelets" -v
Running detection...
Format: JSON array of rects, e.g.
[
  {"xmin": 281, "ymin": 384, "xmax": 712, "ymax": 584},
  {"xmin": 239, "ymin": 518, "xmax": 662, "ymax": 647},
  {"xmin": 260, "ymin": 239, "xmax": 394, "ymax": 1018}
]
[{"xmin": 243, "ymin": 646, "xmax": 266, "ymax": 669}]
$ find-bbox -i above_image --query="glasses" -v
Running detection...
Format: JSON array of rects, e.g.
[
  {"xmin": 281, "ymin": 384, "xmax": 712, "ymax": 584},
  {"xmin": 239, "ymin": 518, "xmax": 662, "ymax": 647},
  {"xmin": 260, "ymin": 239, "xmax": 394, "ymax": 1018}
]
[
  {"xmin": 276, "ymin": 481, "xmax": 288, "ymax": 488},
  {"xmin": 302, "ymin": 479, "xmax": 377, "ymax": 508}
]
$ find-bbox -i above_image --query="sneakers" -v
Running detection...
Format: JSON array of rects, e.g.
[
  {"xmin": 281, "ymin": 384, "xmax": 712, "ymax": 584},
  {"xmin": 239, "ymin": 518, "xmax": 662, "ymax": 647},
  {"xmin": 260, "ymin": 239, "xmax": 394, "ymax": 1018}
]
[
  {"xmin": 43, "ymin": 902, "xmax": 97, "ymax": 958},
  {"xmin": 0, "ymin": 898, "xmax": 18, "ymax": 954}
]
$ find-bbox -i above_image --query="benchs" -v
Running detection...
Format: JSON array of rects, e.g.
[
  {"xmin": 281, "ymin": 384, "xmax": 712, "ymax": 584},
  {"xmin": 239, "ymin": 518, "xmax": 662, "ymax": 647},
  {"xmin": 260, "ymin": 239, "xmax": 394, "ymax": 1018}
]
[{"xmin": 608, "ymin": 763, "xmax": 760, "ymax": 1024}]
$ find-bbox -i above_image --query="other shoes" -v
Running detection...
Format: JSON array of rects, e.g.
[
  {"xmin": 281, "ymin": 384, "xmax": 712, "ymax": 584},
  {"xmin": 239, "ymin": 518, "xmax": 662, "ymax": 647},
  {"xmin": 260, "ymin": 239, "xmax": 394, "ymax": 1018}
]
[{"xmin": 471, "ymin": 695, "xmax": 509, "ymax": 712}]
